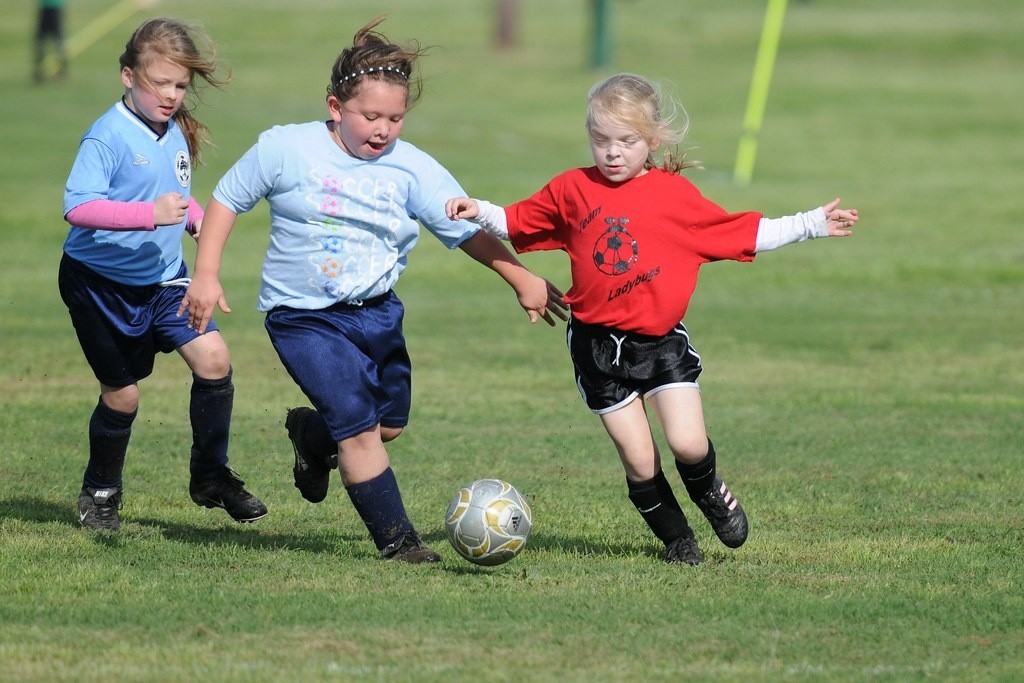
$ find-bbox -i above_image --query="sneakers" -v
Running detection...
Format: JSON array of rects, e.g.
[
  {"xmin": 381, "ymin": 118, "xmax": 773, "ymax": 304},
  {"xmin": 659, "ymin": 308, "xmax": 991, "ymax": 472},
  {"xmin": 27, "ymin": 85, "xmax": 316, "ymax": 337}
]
[
  {"xmin": 697, "ymin": 479, "xmax": 749, "ymax": 548},
  {"xmin": 383, "ymin": 534, "xmax": 441, "ymax": 564},
  {"xmin": 77, "ymin": 487, "xmax": 124, "ymax": 535},
  {"xmin": 664, "ymin": 526, "xmax": 706, "ymax": 567},
  {"xmin": 190, "ymin": 465, "xmax": 269, "ymax": 523},
  {"xmin": 285, "ymin": 407, "xmax": 330, "ymax": 503}
]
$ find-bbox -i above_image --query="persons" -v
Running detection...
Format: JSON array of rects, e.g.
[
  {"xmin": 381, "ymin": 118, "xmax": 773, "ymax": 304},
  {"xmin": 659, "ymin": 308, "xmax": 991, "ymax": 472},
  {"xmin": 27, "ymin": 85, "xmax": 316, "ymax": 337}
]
[
  {"xmin": 445, "ymin": 74, "xmax": 859, "ymax": 565},
  {"xmin": 30, "ymin": 0, "xmax": 72, "ymax": 90},
  {"xmin": 59, "ymin": 19, "xmax": 269, "ymax": 530},
  {"xmin": 176, "ymin": 14, "xmax": 569, "ymax": 565}
]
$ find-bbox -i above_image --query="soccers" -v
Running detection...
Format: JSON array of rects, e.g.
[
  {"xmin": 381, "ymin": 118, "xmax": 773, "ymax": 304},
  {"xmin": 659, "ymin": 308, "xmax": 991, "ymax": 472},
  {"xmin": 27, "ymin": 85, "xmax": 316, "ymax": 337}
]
[{"xmin": 444, "ymin": 478, "xmax": 533, "ymax": 567}]
